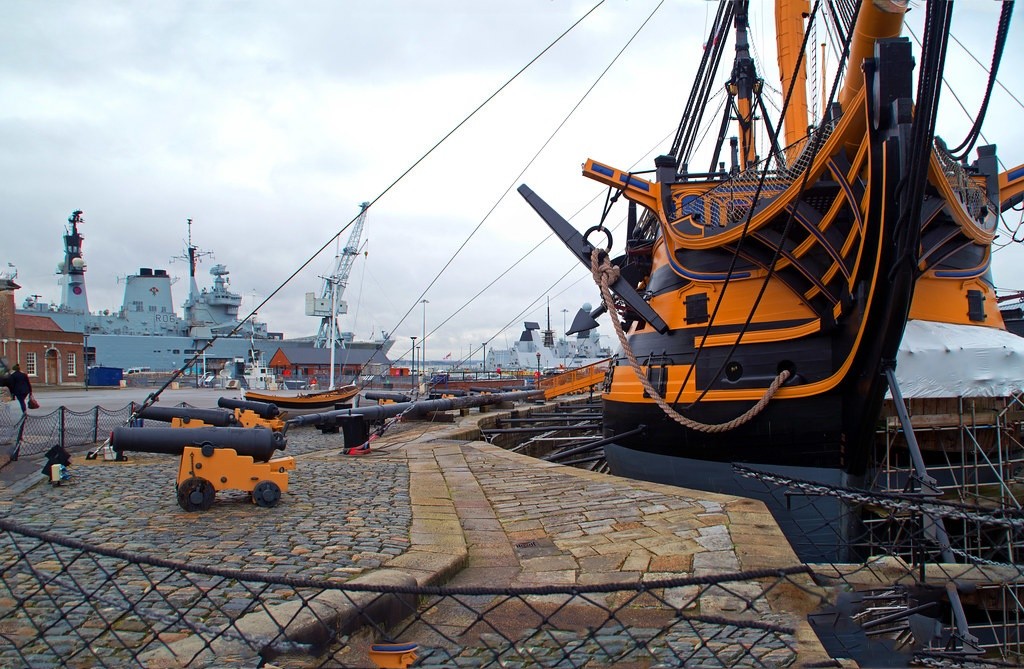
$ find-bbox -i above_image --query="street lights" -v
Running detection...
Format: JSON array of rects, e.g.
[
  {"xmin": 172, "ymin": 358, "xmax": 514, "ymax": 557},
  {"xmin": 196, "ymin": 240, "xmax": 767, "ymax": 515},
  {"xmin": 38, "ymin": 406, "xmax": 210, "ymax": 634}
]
[
  {"xmin": 482, "ymin": 343, "xmax": 487, "ymax": 374},
  {"xmin": 409, "ymin": 336, "xmax": 416, "ymax": 394},
  {"xmin": 536, "ymin": 353, "xmax": 540, "ymax": 389},
  {"xmin": 470, "ymin": 344, "xmax": 472, "ymax": 369},
  {"xmin": 420, "ymin": 299, "xmax": 430, "ymax": 380},
  {"xmin": 560, "ymin": 309, "xmax": 568, "ymax": 370},
  {"xmin": 251, "ymin": 312, "xmax": 258, "ymax": 367}
]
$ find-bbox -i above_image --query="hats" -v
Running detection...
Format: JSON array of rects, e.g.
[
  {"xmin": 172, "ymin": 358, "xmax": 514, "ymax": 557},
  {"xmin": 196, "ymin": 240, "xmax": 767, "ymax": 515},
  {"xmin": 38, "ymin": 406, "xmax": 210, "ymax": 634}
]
[{"xmin": 12, "ymin": 363, "xmax": 21, "ymax": 370}]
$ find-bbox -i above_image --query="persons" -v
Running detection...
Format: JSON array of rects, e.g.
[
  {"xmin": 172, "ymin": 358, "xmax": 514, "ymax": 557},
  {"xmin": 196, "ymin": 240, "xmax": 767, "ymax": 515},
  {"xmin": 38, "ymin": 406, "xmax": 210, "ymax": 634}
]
[{"xmin": 7, "ymin": 364, "xmax": 32, "ymax": 414}]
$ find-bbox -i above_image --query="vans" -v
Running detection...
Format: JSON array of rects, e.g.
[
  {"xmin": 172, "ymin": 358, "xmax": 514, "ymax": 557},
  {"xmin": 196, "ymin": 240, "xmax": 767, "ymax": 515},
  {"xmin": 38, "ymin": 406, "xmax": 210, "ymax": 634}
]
[{"xmin": 123, "ymin": 366, "xmax": 151, "ymax": 375}]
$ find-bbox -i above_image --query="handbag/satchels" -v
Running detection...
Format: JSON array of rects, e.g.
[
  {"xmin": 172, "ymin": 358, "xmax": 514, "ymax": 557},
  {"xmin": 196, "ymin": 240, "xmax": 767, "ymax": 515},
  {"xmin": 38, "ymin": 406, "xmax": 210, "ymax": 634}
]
[{"xmin": 28, "ymin": 394, "xmax": 40, "ymax": 409}]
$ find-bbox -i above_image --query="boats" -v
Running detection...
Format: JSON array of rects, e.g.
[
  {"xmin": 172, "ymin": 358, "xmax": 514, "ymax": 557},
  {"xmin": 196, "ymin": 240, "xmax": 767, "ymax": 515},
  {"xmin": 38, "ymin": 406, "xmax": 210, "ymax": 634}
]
[
  {"xmin": 17, "ymin": 209, "xmax": 395, "ymax": 376},
  {"xmin": 518, "ymin": 0, "xmax": 1024, "ymax": 669}
]
